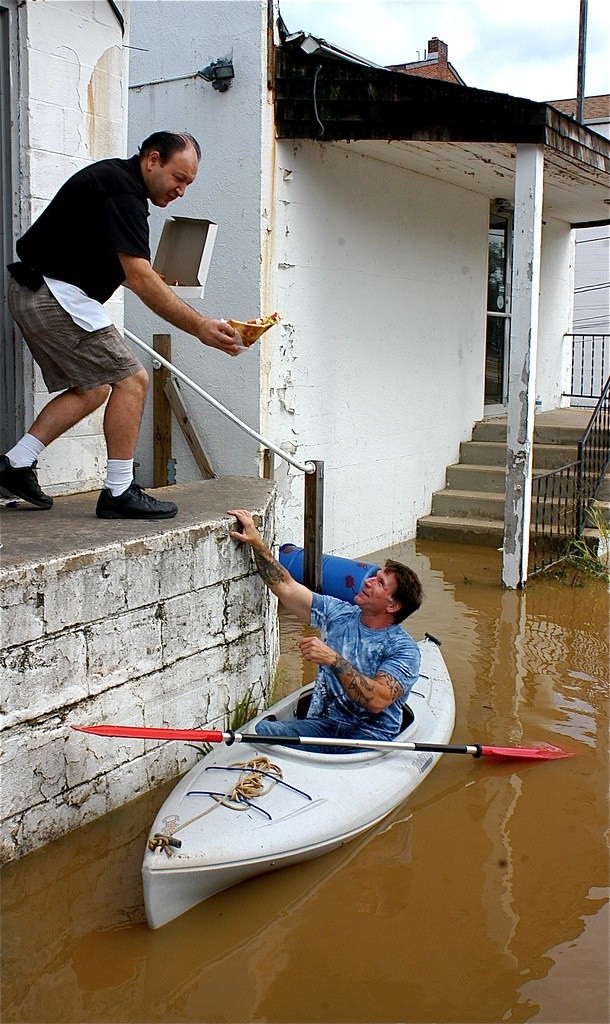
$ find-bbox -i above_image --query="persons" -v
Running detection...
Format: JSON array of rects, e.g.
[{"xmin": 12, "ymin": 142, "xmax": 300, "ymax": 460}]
[
  {"xmin": 0, "ymin": 130, "xmax": 239, "ymax": 521},
  {"xmin": 227, "ymin": 509, "xmax": 424, "ymax": 756}
]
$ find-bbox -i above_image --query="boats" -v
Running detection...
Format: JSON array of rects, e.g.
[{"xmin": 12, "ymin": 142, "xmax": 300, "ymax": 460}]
[{"xmin": 141, "ymin": 633, "xmax": 454, "ymax": 930}]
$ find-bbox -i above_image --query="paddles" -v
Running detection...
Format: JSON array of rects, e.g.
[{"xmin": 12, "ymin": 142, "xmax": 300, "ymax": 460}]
[{"xmin": 68, "ymin": 721, "xmax": 564, "ymax": 759}]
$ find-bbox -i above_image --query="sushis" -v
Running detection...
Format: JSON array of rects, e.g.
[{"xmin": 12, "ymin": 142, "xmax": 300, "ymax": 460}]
[{"xmin": 225, "ymin": 312, "xmax": 281, "ymax": 347}]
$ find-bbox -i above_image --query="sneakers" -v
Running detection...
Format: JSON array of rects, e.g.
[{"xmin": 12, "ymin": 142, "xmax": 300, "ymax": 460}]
[
  {"xmin": 96, "ymin": 483, "xmax": 177, "ymax": 520},
  {"xmin": 0, "ymin": 453, "xmax": 53, "ymax": 509}
]
